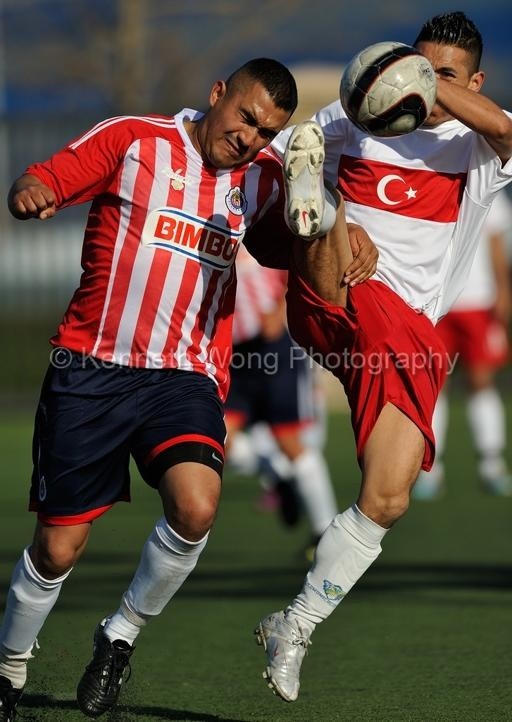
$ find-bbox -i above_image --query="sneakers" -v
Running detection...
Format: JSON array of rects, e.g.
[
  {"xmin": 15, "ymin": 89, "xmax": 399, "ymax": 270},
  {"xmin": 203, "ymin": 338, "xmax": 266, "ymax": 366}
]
[
  {"xmin": 252, "ymin": 608, "xmax": 312, "ymax": 704},
  {"xmin": 0, "ymin": 671, "xmax": 29, "ymax": 721},
  {"xmin": 73, "ymin": 613, "xmax": 138, "ymax": 719},
  {"xmin": 280, "ymin": 117, "xmax": 327, "ymax": 242}
]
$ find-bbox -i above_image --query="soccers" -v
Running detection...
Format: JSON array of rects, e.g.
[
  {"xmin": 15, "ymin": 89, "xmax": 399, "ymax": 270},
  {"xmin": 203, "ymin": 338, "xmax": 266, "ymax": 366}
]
[{"xmin": 339, "ymin": 41, "xmax": 436, "ymax": 136}]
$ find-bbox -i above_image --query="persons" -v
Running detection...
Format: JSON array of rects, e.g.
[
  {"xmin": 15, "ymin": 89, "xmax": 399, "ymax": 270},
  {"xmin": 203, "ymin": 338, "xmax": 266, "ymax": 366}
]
[
  {"xmin": 253, "ymin": 8, "xmax": 511, "ymax": 706},
  {"xmin": 1, "ymin": 55, "xmax": 380, "ymax": 719}
]
